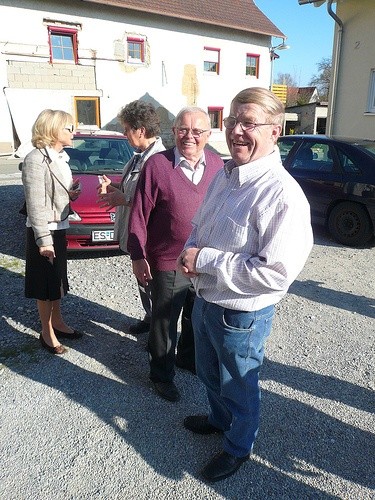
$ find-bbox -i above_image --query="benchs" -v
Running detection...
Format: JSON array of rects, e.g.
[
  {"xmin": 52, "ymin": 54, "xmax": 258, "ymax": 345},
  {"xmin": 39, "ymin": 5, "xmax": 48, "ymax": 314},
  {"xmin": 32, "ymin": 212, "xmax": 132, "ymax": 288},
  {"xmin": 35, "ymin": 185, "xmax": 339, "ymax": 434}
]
[{"xmin": 79, "ymin": 151, "xmax": 110, "ymax": 168}]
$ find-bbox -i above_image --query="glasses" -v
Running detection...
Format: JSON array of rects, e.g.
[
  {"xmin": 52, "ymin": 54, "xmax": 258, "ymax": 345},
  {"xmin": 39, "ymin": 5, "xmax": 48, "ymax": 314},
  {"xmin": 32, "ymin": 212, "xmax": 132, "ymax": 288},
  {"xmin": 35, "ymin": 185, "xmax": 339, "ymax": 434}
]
[
  {"xmin": 223, "ymin": 116, "xmax": 278, "ymax": 132},
  {"xmin": 172, "ymin": 126, "xmax": 212, "ymax": 138},
  {"xmin": 61, "ymin": 125, "xmax": 74, "ymax": 133}
]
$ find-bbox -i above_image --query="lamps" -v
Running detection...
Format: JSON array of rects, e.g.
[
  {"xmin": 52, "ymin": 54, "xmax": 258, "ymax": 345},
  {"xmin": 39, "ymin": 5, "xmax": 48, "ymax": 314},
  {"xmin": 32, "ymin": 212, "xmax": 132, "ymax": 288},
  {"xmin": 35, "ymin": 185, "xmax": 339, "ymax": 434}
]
[{"xmin": 271, "ymin": 38, "xmax": 289, "ymax": 51}]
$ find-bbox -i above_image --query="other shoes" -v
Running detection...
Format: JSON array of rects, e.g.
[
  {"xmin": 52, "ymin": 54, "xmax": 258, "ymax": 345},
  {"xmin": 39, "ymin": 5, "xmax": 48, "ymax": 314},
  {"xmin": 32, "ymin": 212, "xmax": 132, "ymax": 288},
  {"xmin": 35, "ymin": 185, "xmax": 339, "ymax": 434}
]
[
  {"xmin": 39, "ymin": 334, "xmax": 68, "ymax": 354},
  {"xmin": 52, "ymin": 326, "xmax": 82, "ymax": 340},
  {"xmin": 129, "ymin": 314, "xmax": 152, "ymax": 334},
  {"xmin": 151, "ymin": 377, "xmax": 180, "ymax": 400},
  {"xmin": 176, "ymin": 355, "xmax": 197, "ymax": 375}
]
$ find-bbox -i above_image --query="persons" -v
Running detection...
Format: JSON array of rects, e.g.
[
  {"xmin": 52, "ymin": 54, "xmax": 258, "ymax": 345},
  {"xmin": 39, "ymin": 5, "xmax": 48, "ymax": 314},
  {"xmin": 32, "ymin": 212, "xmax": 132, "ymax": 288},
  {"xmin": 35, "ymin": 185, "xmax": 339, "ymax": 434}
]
[
  {"xmin": 23, "ymin": 109, "xmax": 83, "ymax": 354},
  {"xmin": 97, "ymin": 101, "xmax": 169, "ymax": 333},
  {"xmin": 127, "ymin": 106, "xmax": 227, "ymax": 399},
  {"xmin": 176, "ymin": 88, "xmax": 313, "ymax": 478}
]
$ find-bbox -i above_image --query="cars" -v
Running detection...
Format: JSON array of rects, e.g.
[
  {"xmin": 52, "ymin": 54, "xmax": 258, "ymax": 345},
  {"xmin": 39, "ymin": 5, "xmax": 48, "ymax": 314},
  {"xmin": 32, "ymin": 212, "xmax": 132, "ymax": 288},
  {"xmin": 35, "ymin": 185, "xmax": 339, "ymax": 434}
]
[
  {"xmin": 18, "ymin": 130, "xmax": 135, "ymax": 255},
  {"xmin": 276, "ymin": 134, "xmax": 375, "ymax": 247}
]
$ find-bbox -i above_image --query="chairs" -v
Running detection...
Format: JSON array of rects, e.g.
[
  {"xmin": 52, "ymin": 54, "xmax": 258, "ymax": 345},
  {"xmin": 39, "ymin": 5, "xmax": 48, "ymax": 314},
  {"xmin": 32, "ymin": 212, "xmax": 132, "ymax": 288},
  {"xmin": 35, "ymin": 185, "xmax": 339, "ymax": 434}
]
[
  {"xmin": 321, "ymin": 150, "xmax": 334, "ymax": 172},
  {"xmin": 297, "ymin": 148, "xmax": 313, "ymax": 161},
  {"xmin": 94, "ymin": 148, "xmax": 119, "ymax": 169}
]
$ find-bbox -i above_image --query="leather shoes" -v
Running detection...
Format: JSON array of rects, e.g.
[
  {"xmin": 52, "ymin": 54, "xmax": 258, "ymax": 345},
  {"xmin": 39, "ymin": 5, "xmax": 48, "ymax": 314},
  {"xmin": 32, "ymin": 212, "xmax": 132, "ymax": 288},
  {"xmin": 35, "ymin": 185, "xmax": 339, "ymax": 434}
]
[
  {"xmin": 202, "ymin": 451, "xmax": 250, "ymax": 480},
  {"xmin": 183, "ymin": 414, "xmax": 221, "ymax": 434}
]
